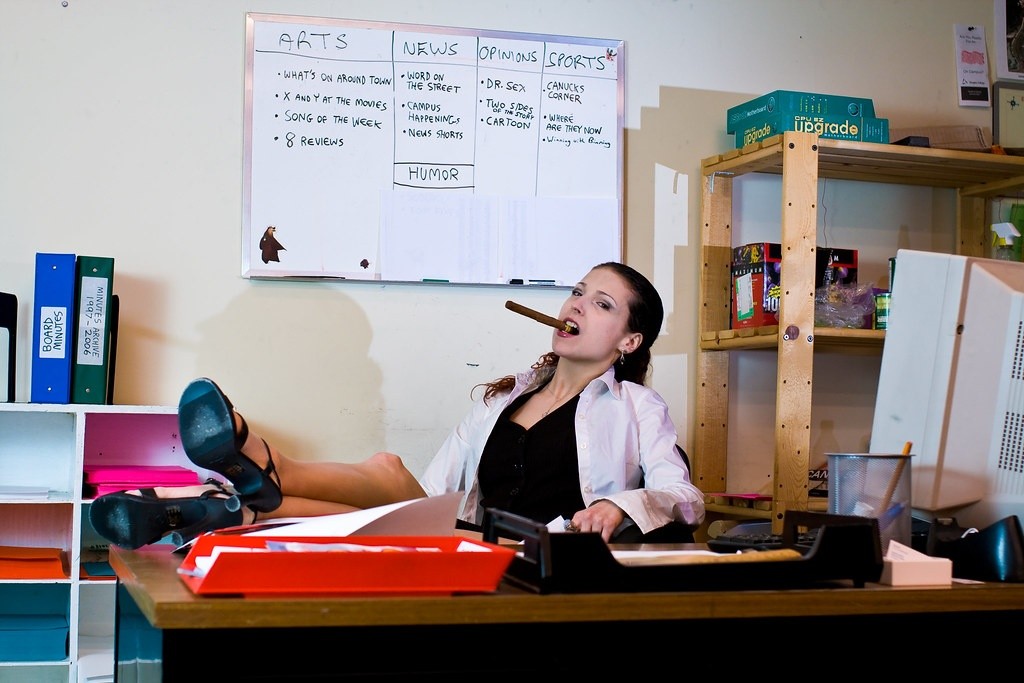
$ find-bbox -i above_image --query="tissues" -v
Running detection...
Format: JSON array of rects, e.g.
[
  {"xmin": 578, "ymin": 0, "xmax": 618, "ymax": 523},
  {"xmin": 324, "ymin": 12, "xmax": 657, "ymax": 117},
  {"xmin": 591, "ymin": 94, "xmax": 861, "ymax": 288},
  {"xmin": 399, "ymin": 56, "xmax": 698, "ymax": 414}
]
[{"xmin": 880, "ymin": 539, "xmax": 953, "ymax": 587}]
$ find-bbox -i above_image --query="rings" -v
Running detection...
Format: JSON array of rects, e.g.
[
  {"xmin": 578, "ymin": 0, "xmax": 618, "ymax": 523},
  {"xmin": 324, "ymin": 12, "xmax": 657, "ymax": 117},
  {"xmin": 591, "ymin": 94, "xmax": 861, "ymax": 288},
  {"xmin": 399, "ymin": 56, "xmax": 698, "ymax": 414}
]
[{"xmin": 563, "ymin": 519, "xmax": 577, "ymax": 531}]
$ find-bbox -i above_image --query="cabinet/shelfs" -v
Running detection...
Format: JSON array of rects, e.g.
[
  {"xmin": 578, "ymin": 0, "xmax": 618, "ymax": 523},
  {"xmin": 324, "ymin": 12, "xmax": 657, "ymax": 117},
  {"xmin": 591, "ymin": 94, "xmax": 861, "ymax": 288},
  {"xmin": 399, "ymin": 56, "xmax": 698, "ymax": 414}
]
[
  {"xmin": 0, "ymin": 401, "xmax": 234, "ymax": 683},
  {"xmin": 691, "ymin": 131, "xmax": 1024, "ymax": 541}
]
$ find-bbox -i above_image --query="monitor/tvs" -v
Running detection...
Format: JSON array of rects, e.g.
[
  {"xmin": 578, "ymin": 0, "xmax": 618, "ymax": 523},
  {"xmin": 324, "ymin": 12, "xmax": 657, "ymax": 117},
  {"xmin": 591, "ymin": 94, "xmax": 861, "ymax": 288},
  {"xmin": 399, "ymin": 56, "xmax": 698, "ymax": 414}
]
[{"xmin": 866, "ymin": 247, "xmax": 1024, "ymax": 533}]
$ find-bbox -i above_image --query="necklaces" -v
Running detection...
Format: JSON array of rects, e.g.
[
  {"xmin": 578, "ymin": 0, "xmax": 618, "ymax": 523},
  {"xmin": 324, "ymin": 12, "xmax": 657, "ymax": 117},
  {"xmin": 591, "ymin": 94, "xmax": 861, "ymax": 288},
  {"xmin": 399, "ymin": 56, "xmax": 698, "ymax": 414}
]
[{"xmin": 542, "ymin": 385, "xmax": 579, "ymax": 419}]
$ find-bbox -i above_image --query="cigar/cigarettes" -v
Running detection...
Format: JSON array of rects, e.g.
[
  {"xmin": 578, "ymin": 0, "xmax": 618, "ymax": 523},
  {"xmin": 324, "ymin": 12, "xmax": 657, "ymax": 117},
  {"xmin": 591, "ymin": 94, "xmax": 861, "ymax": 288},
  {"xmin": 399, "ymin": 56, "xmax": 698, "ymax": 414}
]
[{"xmin": 505, "ymin": 299, "xmax": 580, "ymax": 335}]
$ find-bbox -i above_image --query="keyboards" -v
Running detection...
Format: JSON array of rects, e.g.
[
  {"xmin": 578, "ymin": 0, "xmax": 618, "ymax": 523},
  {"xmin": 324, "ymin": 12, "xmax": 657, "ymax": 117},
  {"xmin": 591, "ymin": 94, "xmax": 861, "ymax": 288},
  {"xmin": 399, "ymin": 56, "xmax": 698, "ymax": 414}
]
[{"xmin": 705, "ymin": 530, "xmax": 819, "ymax": 555}]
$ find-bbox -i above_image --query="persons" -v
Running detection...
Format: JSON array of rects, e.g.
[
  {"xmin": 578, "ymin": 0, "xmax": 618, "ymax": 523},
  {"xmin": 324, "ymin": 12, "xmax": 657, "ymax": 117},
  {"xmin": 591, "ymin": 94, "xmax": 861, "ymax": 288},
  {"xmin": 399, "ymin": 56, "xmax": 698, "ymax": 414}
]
[{"xmin": 89, "ymin": 261, "xmax": 705, "ymax": 544}]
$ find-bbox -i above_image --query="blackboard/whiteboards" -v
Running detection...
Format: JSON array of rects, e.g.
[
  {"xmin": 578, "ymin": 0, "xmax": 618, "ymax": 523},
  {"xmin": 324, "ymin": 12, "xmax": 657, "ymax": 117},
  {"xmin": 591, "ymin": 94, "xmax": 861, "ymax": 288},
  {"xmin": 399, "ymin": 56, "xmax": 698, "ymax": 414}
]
[{"xmin": 239, "ymin": 11, "xmax": 626, "ymax": 293}]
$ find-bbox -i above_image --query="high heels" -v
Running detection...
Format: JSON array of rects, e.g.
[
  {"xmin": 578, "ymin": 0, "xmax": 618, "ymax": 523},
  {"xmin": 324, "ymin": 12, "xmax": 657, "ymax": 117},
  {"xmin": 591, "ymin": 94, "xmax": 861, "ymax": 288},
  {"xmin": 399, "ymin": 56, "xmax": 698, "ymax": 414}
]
[
  {"xmin": 88, "ymin": 477, "xmax": 257, "ymax": 550},
  {"xmin": 178, "ymin": 376, "xmax": 284, "ymax": 513}
]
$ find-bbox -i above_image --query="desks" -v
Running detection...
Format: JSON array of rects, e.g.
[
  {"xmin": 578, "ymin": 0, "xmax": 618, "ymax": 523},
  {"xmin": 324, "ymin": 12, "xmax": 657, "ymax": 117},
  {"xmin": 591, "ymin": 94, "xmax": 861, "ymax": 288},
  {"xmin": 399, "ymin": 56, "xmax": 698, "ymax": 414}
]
[{"xmin": 114, "ymin": 542, "xmax": 1024, "ymax": 683}]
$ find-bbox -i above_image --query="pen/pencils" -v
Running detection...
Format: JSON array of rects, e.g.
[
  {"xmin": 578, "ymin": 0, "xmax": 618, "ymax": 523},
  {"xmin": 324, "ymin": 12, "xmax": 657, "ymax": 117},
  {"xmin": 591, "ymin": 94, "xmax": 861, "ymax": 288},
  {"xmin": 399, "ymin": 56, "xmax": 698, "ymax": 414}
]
[{"xmin": 877, "ymin": 441, "xmax": 914, "ymax": 526}]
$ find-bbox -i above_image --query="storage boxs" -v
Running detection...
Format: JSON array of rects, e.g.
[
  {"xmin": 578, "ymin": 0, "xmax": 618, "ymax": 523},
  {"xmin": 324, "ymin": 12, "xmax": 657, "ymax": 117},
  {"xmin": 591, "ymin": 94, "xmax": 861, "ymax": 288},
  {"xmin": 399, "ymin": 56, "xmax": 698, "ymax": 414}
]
[
  {"xmin": 735, "ymin": 112, "xmax": 891, "ymax": 148},
  {"xmin": 725, "ymin": 89, "xmax": 877, "ymax": 135},
  {"xmin": 729, "ymin": 242, "xmax": 858, "ymax": 328}
]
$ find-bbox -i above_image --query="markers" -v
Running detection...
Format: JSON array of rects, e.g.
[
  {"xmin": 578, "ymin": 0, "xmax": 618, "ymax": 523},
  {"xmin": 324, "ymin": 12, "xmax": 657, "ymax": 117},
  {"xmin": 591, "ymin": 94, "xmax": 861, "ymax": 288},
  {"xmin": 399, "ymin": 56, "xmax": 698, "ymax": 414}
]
[{"xmin": 510, "ymin": 279, "xmax": 557, "ymax": 287}]
